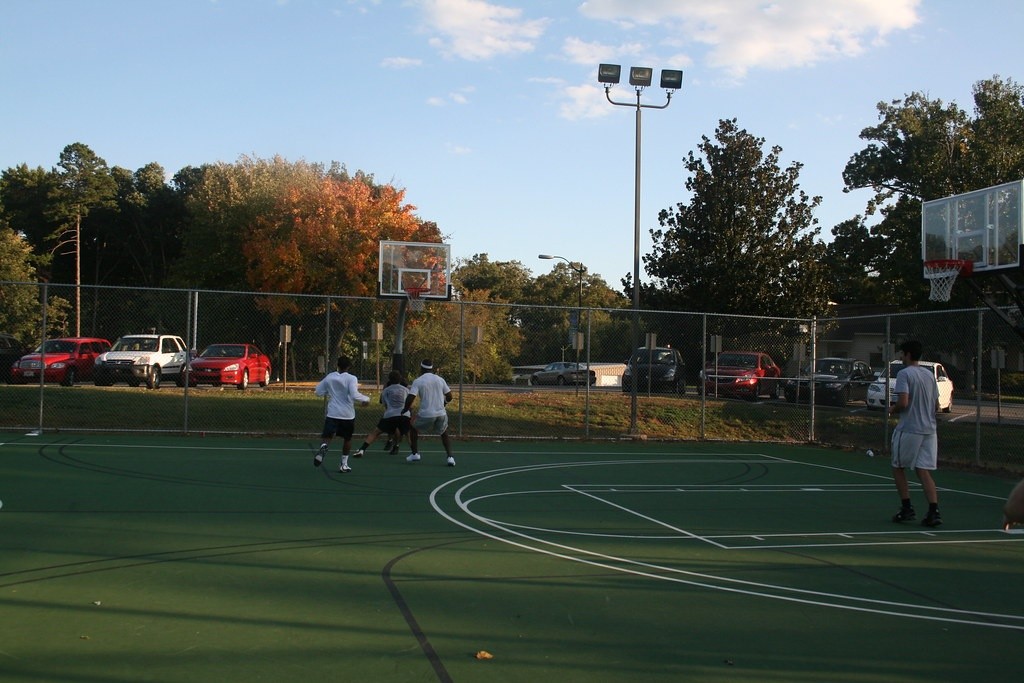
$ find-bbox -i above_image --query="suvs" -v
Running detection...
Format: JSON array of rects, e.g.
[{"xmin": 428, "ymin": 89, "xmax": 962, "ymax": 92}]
[{"xmin": 93, "ymin": 333, "xmax": 190, "ymax": 390}]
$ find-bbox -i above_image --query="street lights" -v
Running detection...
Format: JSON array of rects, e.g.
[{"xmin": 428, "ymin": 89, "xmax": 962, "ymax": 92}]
[
  {"xmin": 595, "ymin": 61, "xmax": 685, "ymax": 436},
  {"xmin": 538, "ymin": 253, "xmax": 588, "ymax": 396}
]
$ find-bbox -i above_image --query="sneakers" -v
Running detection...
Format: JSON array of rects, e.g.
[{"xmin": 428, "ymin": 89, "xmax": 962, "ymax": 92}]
[
  {"xmin": 384, "ymin": 440, "xmax": 394, "ymax": 451},
  {"xmin": 921, "ymin": 510, "xmax": 943, "ymax": 527},
  {"xmin": 447, "ymin": 456, "xmax": 456, "ymax": 467},
  {"xmin": 406, "ymin": 452, "xmax": 421, "ymax": 462},
  {"xmin": 340, "ymin": 464, "xmax": 352, "ymax": 473},
  {"xmin": 314, "ymin": 443, "xmax": 329, "ymax": 467},
  {"xmin": 893, "ymin": 508, "xmax": 916, "ymax": 522},
  {"xmin": 390, "ymin": 444, "xmax": 400, "ymax": 455},
  {"xmin": 353, "ymin": 449, "xmax": 364, "ymax": 457}
]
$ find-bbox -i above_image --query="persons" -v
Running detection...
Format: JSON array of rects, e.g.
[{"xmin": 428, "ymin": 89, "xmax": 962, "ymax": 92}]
[
  {"xmin": 1004, "ymin": 480, "xmax": 1024, "ymax": 532},
  {"xmin": 401, "ymin": 359, "xmax": 457, "ymax": 467},
  {"xmin": 890, "ymin": 340, "xmax": 944, "ymax": 527},
  {"xmin": 314, "ymin": 356, "xmax": 370, "ymax": 474},
  {"xmin": 352, "ymin": 379, "xmax": 413, "ymax": 457},
  {"xmin": 381, "ymin": 371, "xmax": 417, "ymax": 454}
]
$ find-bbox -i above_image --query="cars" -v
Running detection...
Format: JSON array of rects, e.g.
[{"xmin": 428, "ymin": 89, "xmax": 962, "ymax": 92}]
[
  {"xmin": 10, "ymin": 337, "xmax": 112, "ymax": 389},
  {"xmin": 786, "ymin": 358, "xmax": 880, "ymax": 406},
  {"xmin": 866, "ymin": 360, "xmax": 956, "ymax": 415},
  {"xmin": 181, "ymin": 343, "xmax": 272, "ymax": 391},
  {"xmin": 529, "ymin": 361, "xmax": 597, "ymax": 388},
  {"xmin": 621, "ymin": 348, "xmax": 685, "ymax": 394},
  {"xmin": 697, "ymin": 350, "xmax": 780, "ymax": 402}
]
